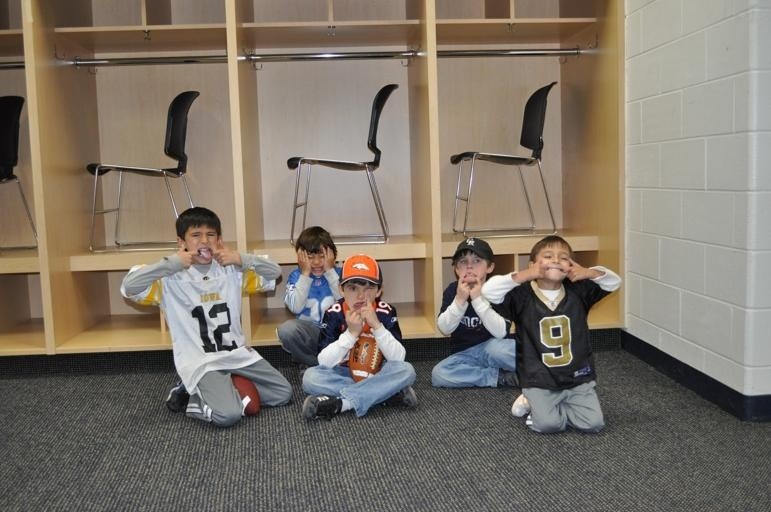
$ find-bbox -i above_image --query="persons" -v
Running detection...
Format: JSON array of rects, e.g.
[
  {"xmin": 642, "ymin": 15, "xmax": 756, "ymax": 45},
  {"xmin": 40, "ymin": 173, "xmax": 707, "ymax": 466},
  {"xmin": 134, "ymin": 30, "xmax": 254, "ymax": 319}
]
[
  {"xmin": 120, "ymin": 207, "xmax": 293, "ymax": 428},
  {"xmin": 480, "ymin": 235, "xmax": 623, "ymax": 435},
  {"xmin": 299, "ymin": 255, "xmax": 418, "ymax": 419},
  {"xmin": 275, "ymin": 225, "xmax": 345, "ymax": 370},
  {"xmin": 431, "ymin": 238, "xmax": 520, "ymax": 390}
]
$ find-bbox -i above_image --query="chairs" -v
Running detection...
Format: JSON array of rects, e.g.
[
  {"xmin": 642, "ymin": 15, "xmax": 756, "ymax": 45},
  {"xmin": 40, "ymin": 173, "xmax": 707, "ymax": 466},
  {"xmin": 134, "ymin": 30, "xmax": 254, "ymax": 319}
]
[
  {"xmin": 0, "ymin": 95, "xmax": 38, "ymax": 251},
  {"xmin": 83, "ymin": 91, "xmax": 204, "ymax": 254},
  {"xmin": 449, "ymin": 79, "xmax": 560, "ymax": 239},
  {"xmin": 287, "ymin": 83, "xmax": 401, "ymax": 246}
]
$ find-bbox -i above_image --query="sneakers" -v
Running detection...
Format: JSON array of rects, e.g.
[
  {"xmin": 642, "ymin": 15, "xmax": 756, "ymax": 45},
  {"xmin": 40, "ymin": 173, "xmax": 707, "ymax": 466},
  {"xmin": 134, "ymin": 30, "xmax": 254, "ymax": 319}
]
[
  {"xmin": 165, "ymin": 379, "xmax": 188, "ymax": 412},
  {"xmin": 497, "ymin": 367, "xmax": 520, "ymax": 388},
  {"xmin": 302, "ymin": 393, "xmax": 342, "ymax": 421},
  {"xmin": 511, "ymin": 393, "xmax": 530, "ymax": 417},
  {"xmin": 382, "ymin": 386, "xmax": 417, "ymax": 407},
  {"xmin": 297, "ymin": 363, "xmax": 308, "ymax": 384}
]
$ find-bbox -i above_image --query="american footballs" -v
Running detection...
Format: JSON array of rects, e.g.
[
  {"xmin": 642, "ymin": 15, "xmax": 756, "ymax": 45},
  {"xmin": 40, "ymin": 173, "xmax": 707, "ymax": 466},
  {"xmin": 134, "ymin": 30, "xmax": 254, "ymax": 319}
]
[
  {"xmin": 230, "ymin": 374, "xmax": 261, "ymax": 416},
  {"xmin": 348, "ymin": 331, "xmax": 381, "ymax": 383}
]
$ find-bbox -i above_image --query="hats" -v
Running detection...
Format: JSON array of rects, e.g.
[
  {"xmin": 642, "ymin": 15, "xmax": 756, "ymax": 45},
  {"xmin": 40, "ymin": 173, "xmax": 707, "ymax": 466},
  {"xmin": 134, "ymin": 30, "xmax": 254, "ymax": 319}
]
[
  {"xmin": 452, "ymin": 237, "xmax": 494, "ymax": 262},
  {"xmin": 339, "ymin": 255, "xmax": 382, "ymax": 285}
]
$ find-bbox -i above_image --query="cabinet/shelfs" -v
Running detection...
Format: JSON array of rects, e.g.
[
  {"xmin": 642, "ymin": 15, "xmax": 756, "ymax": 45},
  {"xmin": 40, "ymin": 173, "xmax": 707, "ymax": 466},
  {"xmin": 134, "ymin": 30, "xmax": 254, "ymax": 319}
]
[{"xmin": 1, "ymin": 0, "xmax": 628, "ymax": 358}]
